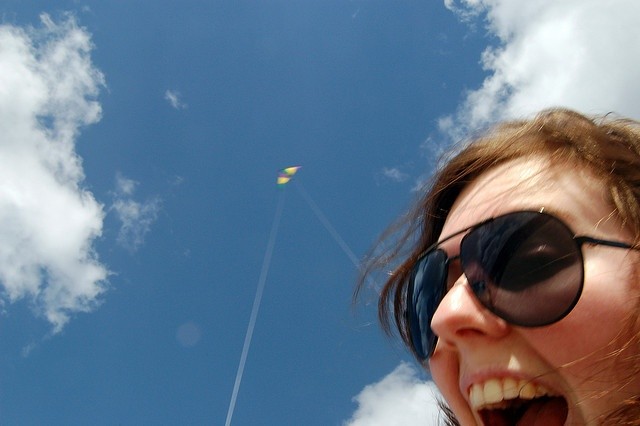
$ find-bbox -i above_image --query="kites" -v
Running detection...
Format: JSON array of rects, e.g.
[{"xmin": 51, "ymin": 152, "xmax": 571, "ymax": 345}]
[{"xmin": 273, "ymin": 163, "xmax": 303, "ymax": 188}]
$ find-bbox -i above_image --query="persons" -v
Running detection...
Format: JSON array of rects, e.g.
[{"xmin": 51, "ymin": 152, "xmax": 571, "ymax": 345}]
[{"xmin": 353, "ymin": 105, "xmax": 640, "ymax": 425}]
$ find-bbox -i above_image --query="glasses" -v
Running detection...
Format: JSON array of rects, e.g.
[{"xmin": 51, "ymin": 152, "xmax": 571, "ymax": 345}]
[{"xmin": 405, "ymin": 209, "xmax": 640, "ymax": 365}]
